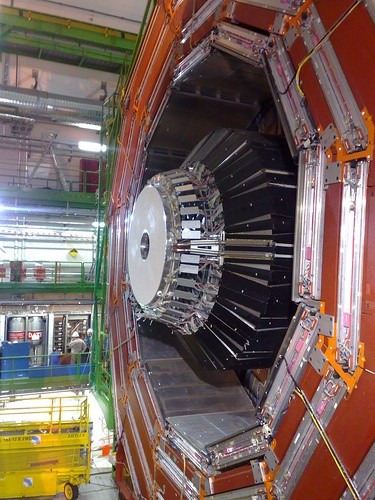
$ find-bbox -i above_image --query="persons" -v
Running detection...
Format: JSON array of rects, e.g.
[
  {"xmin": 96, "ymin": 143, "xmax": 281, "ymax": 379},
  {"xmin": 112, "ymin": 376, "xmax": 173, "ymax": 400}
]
[{"xmin": 67, "ymin": 331, "xmax": 87, "ymax": 364}]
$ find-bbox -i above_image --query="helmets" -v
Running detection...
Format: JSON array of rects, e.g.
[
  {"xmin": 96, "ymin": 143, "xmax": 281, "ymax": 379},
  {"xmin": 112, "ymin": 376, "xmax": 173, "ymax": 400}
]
[{"xmin": 71, "ymin": 332, "xmax": 80, "ymax": 338}]
